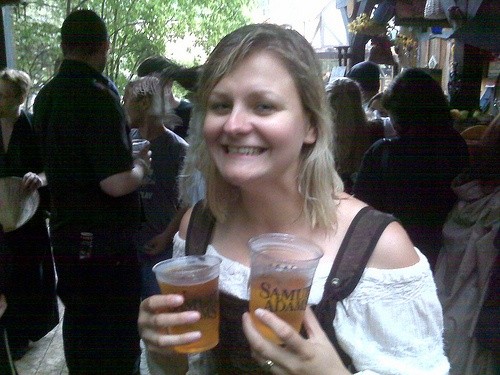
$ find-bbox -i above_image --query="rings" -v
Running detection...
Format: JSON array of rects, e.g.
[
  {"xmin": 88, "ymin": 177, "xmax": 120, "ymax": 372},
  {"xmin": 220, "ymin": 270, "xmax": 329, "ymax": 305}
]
[{"xmin": 263, "ymin": 360, "xmax": 274, "ymax": 370}]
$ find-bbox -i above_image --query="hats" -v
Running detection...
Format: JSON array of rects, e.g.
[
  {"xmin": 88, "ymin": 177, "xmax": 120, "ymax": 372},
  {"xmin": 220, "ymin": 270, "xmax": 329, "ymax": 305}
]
[{"xmin": 0, "ymin": 176, "xmax": 41, "ymax": 231}]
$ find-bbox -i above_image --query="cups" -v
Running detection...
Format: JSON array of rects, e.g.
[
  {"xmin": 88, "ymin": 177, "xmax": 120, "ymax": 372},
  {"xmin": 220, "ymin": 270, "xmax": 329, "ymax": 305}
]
[
  {"xmin": 247, "ymin": 233, "xmax": 324, "ymax": 345},
  {"xmin": 132, "ymin": 139, "xmax": 147, "ymax": 157},
  {"xmin": 152, "ymin": 255, "xmax": 223, "ymax": 354}
]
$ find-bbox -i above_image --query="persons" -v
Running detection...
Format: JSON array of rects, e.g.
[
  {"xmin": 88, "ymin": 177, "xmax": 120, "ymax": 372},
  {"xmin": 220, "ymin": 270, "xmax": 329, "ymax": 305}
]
[
  {"xmin": 138, "ymin": 24, "xmax": 451, "ymax": 375},
  {"xmin": 365, "ymin": 90, "xmax": 396, "ymax": 139},
  {"xmin": 465, "ymin": 75, "xmax": 500, "ymax": 153},
  {"xmin": 126, "ymin": 55, "xmax": 197, "ymax": 156},
  {"xmin": 429, "ymin": 136, "xmax": 499, "ymax": 375},
  {"xmin": 33, "ymin": 9, "xmax": 154, "ymax": 375},
  {"xmin": 117, "ymin": 76, "xmax": 191, "ymax": 375},
  {"xmin": 351, "ymin": 67, "xmax": 472, "ymax": 277},
  {"xmin": 0, "ymin": 69, "xmax": 60, "ymax": 363},
  {"xmin": 345, "ymin": 62, "xmax": 381, "ymax": 114},
  {"xmin": 321, "ymin": 76, "xmax": 398, "ymax": 194}
]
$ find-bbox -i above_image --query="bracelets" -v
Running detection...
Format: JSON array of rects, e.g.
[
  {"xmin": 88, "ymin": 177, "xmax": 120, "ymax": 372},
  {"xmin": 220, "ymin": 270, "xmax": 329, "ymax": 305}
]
[{"xmin": 135, "ymin": 158, "xmax": 149, "ymax": 177}]
